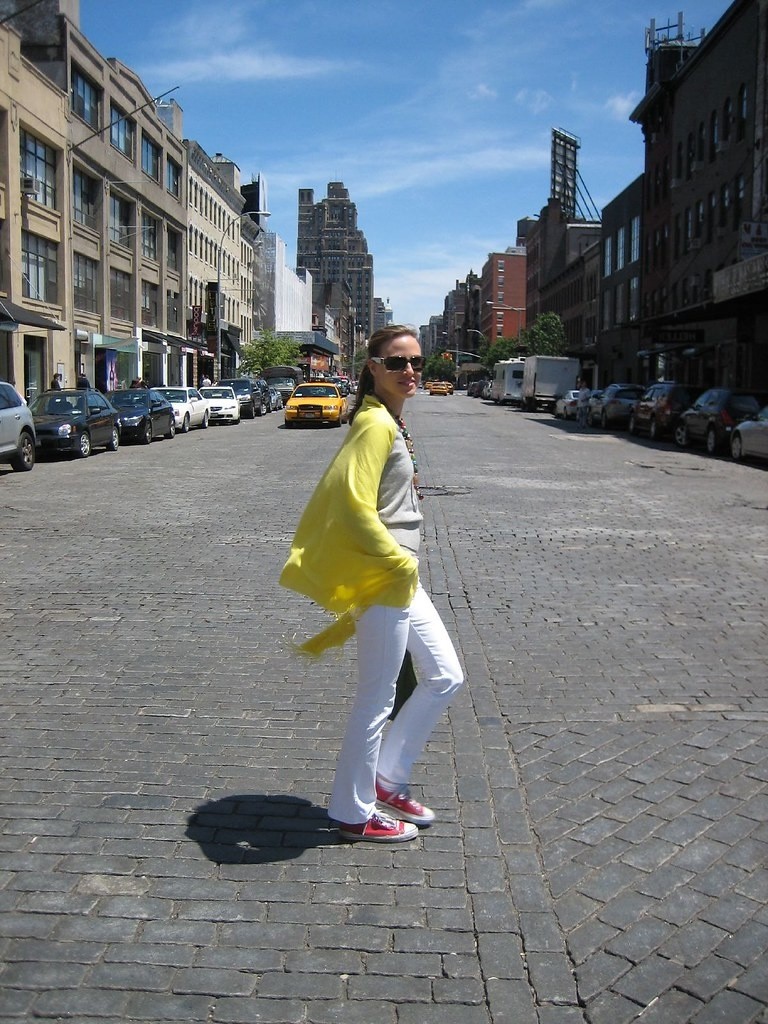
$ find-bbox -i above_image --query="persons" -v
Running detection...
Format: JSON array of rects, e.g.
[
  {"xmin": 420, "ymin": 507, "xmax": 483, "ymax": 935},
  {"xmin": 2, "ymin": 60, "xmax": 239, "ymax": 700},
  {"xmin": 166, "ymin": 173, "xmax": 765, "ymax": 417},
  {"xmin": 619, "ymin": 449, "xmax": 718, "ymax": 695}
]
[
  {"xmin": 278, "ymin": 323, "xmax": 464, "ymax": 843},
  {"xmin": 198, "ymin": 374, "xmax": 211, "ymax": 389},
  {"xmin": 51, "ymin": 373, "xmax": 62, "ymax": 391},
  {"xmin": 76, "ymin": 372, "xmax": 91, "ymax": 391},
  {"xmin": 576, "ymin": 379, "xmax": 591, "ymax": 430}
]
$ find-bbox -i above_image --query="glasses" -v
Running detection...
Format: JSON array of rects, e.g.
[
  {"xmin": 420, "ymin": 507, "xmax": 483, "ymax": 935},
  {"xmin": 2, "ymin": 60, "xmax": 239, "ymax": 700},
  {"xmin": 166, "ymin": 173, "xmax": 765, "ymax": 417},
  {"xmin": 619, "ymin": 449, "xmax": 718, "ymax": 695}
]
[{"xmin": 371, "ymin": 355, "xmax": 426, "ymax": 373}]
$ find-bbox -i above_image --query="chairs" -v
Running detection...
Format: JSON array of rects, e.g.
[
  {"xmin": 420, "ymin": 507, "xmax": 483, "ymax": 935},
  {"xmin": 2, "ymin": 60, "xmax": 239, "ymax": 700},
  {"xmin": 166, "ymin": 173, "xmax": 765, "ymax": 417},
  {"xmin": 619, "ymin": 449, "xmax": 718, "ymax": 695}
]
[
  {"xmin": 165, "ymin": 396, "xmax": 172, "ymax": 400},
  {"xmin": 204, "ymin": 392, "xmax": 212, "ymax": 398},
  {"xmin": 54, "ymin": 401, "xmax": 72, "ymax": 413}
]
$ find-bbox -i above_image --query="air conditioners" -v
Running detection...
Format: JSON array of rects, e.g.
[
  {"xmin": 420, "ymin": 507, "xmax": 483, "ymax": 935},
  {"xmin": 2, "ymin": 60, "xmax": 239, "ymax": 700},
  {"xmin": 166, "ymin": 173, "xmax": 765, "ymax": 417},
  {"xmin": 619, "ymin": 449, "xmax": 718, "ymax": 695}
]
[
  {"xmin": 686, "ymin": 239, "xmax": 701, "ymax": 250},
  {"xmin": 20, "ymin": 177, "xmax": 41, "ymax": 195},
  {"xmin": 690, "ymin": 161, "xmax": 703, "ymax": 173},
  {"xmin": 716, "ymin": 140, "xmax": 728, "ymax": 153}
]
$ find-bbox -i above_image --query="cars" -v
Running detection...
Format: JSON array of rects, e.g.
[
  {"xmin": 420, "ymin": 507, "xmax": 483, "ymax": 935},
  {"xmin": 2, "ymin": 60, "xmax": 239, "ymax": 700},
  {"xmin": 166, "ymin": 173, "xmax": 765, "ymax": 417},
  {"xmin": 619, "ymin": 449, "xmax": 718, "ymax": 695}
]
[
  {"xmin": 254, "ymin": 379, "xmax": 284, "ymax": 415},
  {"xmin": 197, "ymin": 386, "xmax": 242, "ymax": 426},
  {"xmin": 554, "ymin": 381, "xmax": 647, "ymax": 430},
  {"xmin": 628, "ymin": 385, "xmax": 705, "ymax": 442},
  {"xmin": 284, "ymin": 384, "xmax": 348, "ymax": 429},
  {"xmin": 28, "ymin": 387, "xmax": 122, "ymax": 459},
  {"xmin": 323, "ymin": 374, "xmax": 360, "ymax": 396},
  {"xmin": 214, "ymin": 379, "xmax": 265, "ymax": 420},
  {"xmin": 729, "ymin": 406, "xmax": 768, "ymax": 463},
  {"xmin": 103, "ymin": 388, "xmax": 176, "ymax": 445},
  {"xmin": 0, "ymin": 381, "xmax": 38, "ymax": 472},
  {"xmin": 149, "ymin": 386, "xmax": 211, "ymax": 434},
  {"xmin": 672, "ymin": 387, "xmax": 768, "ymax": 455},
  {"xmin": 416, "ymin": 380, "xmax": 492, "ymax": 400}
]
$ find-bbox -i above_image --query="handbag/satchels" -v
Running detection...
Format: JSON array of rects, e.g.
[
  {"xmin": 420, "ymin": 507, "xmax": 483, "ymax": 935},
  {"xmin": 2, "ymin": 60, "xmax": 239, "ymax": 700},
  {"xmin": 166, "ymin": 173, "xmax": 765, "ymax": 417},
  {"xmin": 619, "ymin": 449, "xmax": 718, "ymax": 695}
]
[{"xmin": 385, "ymin": 650, "xmax": 417, "ymax": 721}]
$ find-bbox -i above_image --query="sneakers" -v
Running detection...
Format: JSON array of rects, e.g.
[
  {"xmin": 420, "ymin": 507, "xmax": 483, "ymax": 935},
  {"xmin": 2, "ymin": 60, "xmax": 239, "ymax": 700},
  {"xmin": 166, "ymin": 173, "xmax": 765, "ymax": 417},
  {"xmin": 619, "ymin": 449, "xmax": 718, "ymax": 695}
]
[
  {"xmin": 375, "ymin": 777, "xmax": 435, "ymax": 825},
  {"xmin": 339, "ymin": 810, "xmax": 418, "ymax": 843}
]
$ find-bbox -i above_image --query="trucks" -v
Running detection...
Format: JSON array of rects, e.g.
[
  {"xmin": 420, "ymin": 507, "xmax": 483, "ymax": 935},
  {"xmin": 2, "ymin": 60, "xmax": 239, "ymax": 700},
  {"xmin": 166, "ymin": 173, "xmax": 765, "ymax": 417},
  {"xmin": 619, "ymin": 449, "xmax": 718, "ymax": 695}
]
[
  {"xmin": 263, "ymin": 366, "xmax": 303, "ymax": 401},
  {"xmin": 491, "ymin": 359, "xmax": 525, "ymax": 406},
  {"xmin": 517, "ymin": 356, "xmax": 578, "ymax": 411}
]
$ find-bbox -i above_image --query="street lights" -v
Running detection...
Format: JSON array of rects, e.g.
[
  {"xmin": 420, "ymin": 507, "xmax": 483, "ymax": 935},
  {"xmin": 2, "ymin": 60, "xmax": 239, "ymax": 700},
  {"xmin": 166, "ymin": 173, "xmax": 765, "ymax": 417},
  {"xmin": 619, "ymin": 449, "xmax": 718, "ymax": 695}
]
[
  {"xmin": 217, "ymin": 210, "xmax": 271, "ymax": 381},
  {"xmin": 486, "ymin": 301, "xmax": 521, "ymax": 339}
]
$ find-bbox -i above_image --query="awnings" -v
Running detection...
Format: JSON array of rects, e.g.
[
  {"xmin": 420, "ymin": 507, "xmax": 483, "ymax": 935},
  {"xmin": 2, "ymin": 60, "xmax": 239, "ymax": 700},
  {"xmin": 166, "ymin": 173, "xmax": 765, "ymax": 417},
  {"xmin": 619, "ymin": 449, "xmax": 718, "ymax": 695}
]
[
  {"xmin": 0, "ymin": 248, "xmax": 67, "ymax": 332},
  {"xmin": 636, "ymin": 342, "xmax": 690, "ymax": 370},
  {"xmin": 142, "ymin": 329, "xmax": 208, "ymax": 351},
  {"xmin": 223, "ymin": 330, "xmax": 247, "ymax": 359}
]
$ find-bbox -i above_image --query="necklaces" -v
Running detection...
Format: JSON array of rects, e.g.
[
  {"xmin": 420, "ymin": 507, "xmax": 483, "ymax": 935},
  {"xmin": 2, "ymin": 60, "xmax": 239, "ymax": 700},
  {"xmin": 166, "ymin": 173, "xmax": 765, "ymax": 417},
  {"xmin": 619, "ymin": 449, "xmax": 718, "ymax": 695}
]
[{"xmin": 368, "ymin": 389, "xmax": 424, "ymax": 500}]
